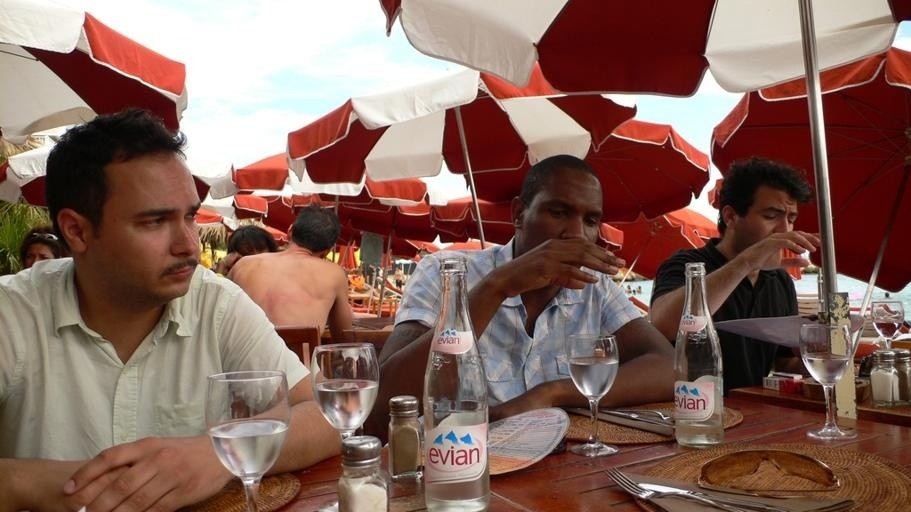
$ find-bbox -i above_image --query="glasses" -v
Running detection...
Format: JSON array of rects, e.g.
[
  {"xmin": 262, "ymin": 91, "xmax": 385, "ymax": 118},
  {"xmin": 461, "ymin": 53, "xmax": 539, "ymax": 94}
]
[
  {"xmin": 695, "ymin": 449, "xmax": 842, "ymax": 500},
  {"xmin": 27, "ymin": 232, "xmax": 60, "ymax": 244}
]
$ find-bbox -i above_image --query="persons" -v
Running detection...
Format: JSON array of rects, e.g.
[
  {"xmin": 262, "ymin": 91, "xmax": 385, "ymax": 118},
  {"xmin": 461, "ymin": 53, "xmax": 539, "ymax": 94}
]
[
  {"xmin": 368, "ymin": 152, "xmax": 694, "ymax": 447},
  {"xmin": 217, "ymin": 206, "xmax": 352, "ymax": 347},
  {"xmin": 652, "ymin": 152, "xmax": 822, "ymax": 393},
  {"xmin": 17, "ymin": 221, "xmax": 69, "ymax": 271},
  {"xmin": 0, "ymin": 105, "xmax": 347, "ymax": 512}
]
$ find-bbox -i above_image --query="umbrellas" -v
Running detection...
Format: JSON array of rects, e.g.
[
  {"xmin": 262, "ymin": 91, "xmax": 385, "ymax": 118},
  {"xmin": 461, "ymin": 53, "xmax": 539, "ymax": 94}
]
[
  {"xmin": 1, "ymin": 4, "xmax": 191, "ymax": 136},
  {"xmin": 0, "ymin": 125, "xmax": 241, "ymax": 226}
]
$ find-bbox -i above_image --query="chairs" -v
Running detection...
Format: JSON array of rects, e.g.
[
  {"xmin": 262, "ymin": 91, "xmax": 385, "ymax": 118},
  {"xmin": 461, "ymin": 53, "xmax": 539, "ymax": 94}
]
[{"xmin": 273, "ymin": 326, "xmax": 392, "ymax": 367}]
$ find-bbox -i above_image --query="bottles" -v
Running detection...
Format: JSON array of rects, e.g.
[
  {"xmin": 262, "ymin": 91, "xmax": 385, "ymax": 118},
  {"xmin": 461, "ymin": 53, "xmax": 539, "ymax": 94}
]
[
  {"xmin": 387, "ymin": 396, "xmax": 423, "ymax": 480},
  {"xmin": 336, "ymin": 435, "xmax": 390, "ymax": 512},
  {"xmin": 670, "ymin": 262, "xmax": 725, "ymax": 450},
  {"xmin": 870, "ymin": 349, "xmax": 911, "ymax": 409},
  {"xmin": 423, "ymin": 255, "xmax": 492, "ymax": 512}
]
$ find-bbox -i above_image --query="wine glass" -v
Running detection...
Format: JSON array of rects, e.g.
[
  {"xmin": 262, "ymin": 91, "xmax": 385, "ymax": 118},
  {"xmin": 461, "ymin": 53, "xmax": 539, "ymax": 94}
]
[
  {"xmin": 870, "ymin": 301, "xmax": 905, "ymax": 350},
  {"xmin": 310, "ymin": 341, "xmax": 380, "ymax": 512},
  {"xmin": 799, "ymin": 323, "xmax": 858, "ymax": 441},
  {"xmin": 567, "ymin": 332, "xmax": 620, "ymax": 457},
  {"xmin": 206, "ymin": 370, "xmax": 291, "ymax": 512}
]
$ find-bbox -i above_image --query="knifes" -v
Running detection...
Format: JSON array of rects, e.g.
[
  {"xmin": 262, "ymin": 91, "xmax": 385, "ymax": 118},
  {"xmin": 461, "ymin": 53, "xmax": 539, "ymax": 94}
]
[
  {"xmin": 600, "ymin": 408, "xmax": 676, "ymax": 431},
  {"xmin": 638, "ymin": 480, "xmax": 796, "ymax": 512}
]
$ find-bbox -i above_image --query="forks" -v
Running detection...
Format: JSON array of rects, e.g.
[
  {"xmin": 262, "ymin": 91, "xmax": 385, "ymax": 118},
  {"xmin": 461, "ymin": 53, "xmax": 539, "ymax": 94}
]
[{"xmin": 602, "ymin": 465, "xmax": 746, "ymax": 512}]
